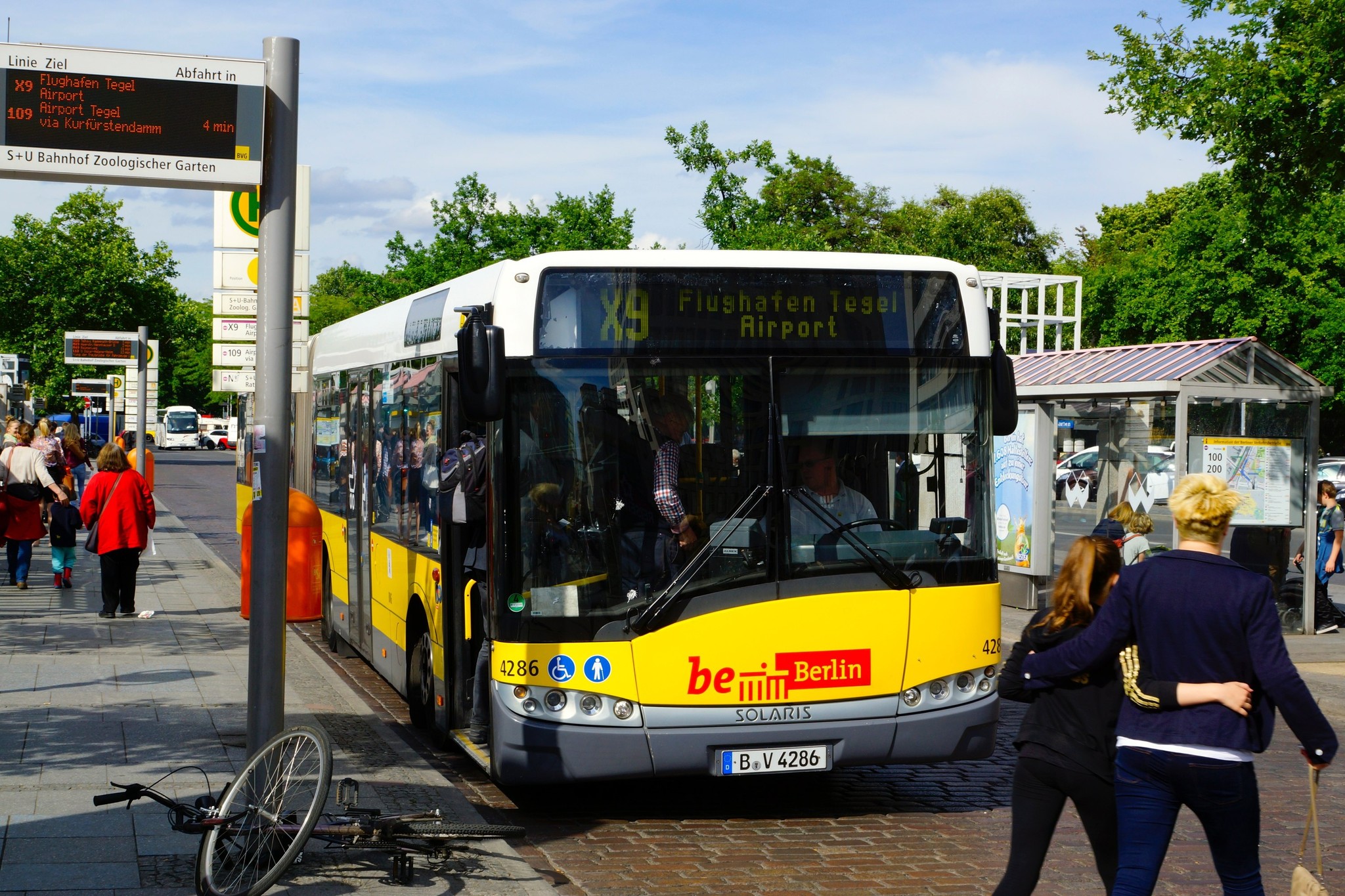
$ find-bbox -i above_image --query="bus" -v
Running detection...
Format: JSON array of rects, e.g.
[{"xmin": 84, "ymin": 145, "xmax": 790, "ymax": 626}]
[
  {"xmin": 153, "ymin": 405, "xmax": 203, "ymax": 451},
  {"xmin": 235, "ymin": 246, "xmax": 1020, "ymax": 807}
]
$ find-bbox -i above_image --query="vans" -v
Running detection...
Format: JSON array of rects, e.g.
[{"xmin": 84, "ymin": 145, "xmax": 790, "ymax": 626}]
[
  {"xmin": 227, "ymin": 417, "xmax": 238, "ymax": 447},
  {"xmin": 47, "ymin": 414, "xmax": 119, "ymax": 446}
]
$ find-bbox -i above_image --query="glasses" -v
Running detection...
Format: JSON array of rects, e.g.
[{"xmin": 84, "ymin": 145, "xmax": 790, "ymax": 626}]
[
  {"xmin": 7, "ymin": 418, "xmax": 16, "ymax": 428},
  {"xmin": 427, "ymin": 422, "xmax": 433, "ymax": 429}
]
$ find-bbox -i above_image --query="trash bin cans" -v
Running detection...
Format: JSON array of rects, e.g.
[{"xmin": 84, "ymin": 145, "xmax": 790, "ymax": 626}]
[
  {"xmin": 127, "ymin": 447, "xmax": 154, "ymax": 492},
  {"xmin": 241, "ymin": 488, "xmax": 324, "ymax": 623},
  {"xmin": 115, "ymin": 436, "xmax": 125, "ymax": 454}
]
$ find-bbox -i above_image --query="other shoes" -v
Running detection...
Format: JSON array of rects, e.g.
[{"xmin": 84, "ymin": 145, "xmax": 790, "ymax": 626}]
[
  {"xmin": 376, "ymin": 511, "xmax": 391, "ymax": 521},
  {"xmin": 17, "ymin": 582, "xmax": 27, "ymax": 589},
  {"xmin": 9, "ymin": 578, "xmax": 17, "ymax": 585}
]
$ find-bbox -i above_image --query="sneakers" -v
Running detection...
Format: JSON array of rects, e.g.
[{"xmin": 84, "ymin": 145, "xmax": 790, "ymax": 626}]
[
  {"xmin": 420, "ymin": 532, "xmax": 429, "ymax": 542},
  {"xmin": 1316, "ymin": 618, "xmax": 1339, "ymax": 635},
  {"xmin": 469, "ymin": 722, "xmax": 490, "ymax": 742}
]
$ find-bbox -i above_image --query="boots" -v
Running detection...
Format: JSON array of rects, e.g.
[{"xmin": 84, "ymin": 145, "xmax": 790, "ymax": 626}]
[
  {"xmin": 63, "ymin": 567, "xmax": 73, "ymax": 588},
  {"xmin": 54, "ymin": 572, "xmax": 62, "ymax": 588}
]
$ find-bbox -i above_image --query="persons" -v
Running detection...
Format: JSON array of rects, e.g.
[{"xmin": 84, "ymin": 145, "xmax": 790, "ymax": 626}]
[
  {"xmin": 619, "ymin": 389, "xmax": 698, "ymax": 603},
  {"xmin": 759, "ymin": 438, "xmax": 884, "ymax": 536},
  {"xmin": 467, "ymin": 381, "xmax": 560, "ymax": 743},
  {"xmin": 992, "ymin": 472, "xmax": 1339, "ymax": 896},
  {"xmin": 0, "ymin": 416, "xmax": 94, "ymax": 588},
  {"xmin": 313, "ymin": 416, "xmax": 442, "ymax": 538},
  {"xmin": 1293, "ymin": 480, "xmax": 1345, "ymax": 634},
  {"xmin": 520, "ymin": 483, "xmax": 568, "ymax": 586},
  {"xmin": 79, "ymin": 443, "xmax": 157, "ymax": 619},
  {"xmin": 895, "ymin": 452, "xmax": 918, "ymax": 531},
  {"xmin": 732, "ymin": 449, "xmax": 741, "ymax": 475}
]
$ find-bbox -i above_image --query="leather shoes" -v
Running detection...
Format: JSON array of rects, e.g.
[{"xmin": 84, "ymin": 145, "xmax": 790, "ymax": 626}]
[
  {"xmin": 99, "ymin": 610, "xmax": 115, "ymax": 618},
  {"xmin": 120, "ymin": 606, "xmax": 135, "ymax": 613}
]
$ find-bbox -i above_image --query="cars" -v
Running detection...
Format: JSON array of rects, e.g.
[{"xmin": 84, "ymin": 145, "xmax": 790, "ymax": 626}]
[
  {"xmin": 217, "ymin": 437, "xmax": 237, "ymax": 451},
  {"xmin": 1054, "ymin": 442, "xmax": 1176, "ymax": 506},
  {"xmin": 84, "ymin": 431, "xmax": 107, "ymax": 459},
  {"xmin": 1301, "ymin": 456, "xmax": 1345, "ymax": 518},
  {"xmin": 203, "ymin": 429, "xmax": 229, "ymax": 450}
]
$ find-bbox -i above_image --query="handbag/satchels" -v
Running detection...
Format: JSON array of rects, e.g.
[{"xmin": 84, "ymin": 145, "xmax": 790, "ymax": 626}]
[
  {"xmin": 1288, "ymin": 866, "xmax": 1331, "ymax": 896},
  {"xmin": 62, "ymin": 473, "xmax": 77, "ymax": 501},
  {"xmin": 84, "ymin": 520, "xmax": 100, "ymax": 554},
  {"xmin": 421, "ymin": 464, "xmax": 440, "ymax": 490}
]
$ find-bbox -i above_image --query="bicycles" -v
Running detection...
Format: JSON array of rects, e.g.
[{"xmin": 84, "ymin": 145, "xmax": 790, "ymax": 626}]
[{"xmin": 93, "ymin": 726, "xmax": 528, "ymax": 895}]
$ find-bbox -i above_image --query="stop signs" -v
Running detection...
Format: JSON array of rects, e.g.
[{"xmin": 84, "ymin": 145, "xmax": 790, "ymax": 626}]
[{"xmin": 83, "ymin": 397, "xmax": 92, "ymax": 409}]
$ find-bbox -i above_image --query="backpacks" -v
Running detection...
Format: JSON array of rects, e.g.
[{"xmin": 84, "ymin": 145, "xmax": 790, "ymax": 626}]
[
  {"xmin": 440, "ymin": 428, "xmax": 493, "ymax": 529},
  {"xmin": 1113, "ymin": 533, "xmax": 1142, "ymax": 566}
]
